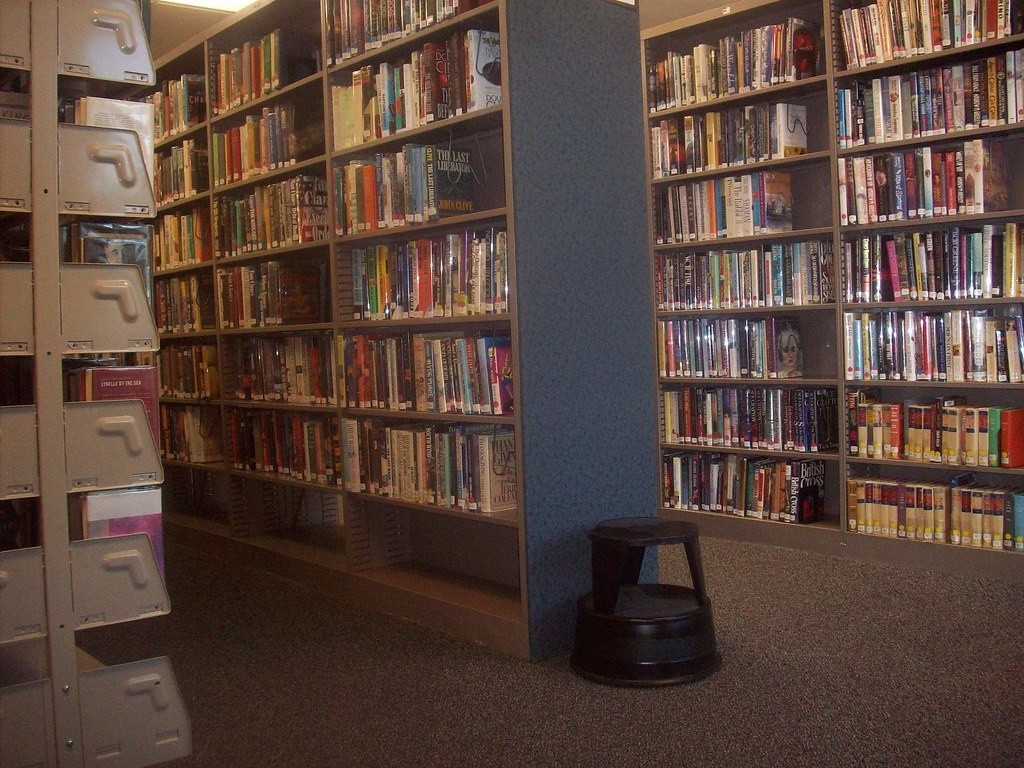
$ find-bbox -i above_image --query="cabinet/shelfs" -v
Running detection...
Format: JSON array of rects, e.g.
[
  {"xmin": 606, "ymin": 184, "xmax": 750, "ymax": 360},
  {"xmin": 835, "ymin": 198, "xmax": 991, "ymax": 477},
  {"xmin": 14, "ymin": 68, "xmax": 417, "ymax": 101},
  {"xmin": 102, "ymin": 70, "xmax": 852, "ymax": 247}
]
[{"xmin": 0, "ymin": 0, "xmax": 1023, "ymax": 767}]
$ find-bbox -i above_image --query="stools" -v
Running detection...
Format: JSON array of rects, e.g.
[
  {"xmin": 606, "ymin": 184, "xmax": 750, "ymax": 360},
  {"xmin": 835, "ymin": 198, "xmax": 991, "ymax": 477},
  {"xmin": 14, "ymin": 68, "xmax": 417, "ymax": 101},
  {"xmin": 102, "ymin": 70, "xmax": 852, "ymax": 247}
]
[{"xmin": 571, "ymin": 515, "xmax": 720, "ymax": 687}]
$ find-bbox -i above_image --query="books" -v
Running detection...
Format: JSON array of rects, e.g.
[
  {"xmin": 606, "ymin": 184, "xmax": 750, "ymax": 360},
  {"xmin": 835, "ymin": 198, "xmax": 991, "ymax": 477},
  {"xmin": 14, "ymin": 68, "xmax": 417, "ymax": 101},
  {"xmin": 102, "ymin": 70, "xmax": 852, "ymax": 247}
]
[
  {"xmin": 0, "ymin": 88, "xmax": 165, "ymax": 585},
  {"xmin": 647, "ymin": 0, "xmax": 1024, "ymax": 552},
  {"xmin": 122, "ymin": 0, "xmax": 520, "ymax": 512}
]
